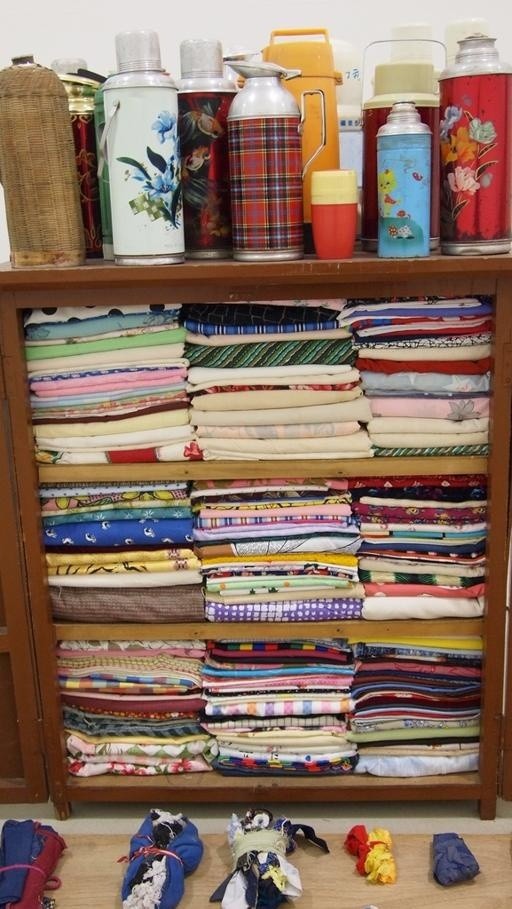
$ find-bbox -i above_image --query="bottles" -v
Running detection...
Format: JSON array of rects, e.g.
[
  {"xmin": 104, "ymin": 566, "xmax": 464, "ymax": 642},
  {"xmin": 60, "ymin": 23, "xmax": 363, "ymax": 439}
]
[{"xmin": 376, "ymin": 101, "xmax": 432, "ymax": 258}]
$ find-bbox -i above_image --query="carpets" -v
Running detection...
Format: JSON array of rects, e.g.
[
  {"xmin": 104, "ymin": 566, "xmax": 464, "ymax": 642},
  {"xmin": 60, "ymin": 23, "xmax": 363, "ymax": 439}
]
[{"xmin": 44, "ymin": 831, "xmax": 512, "ymax": 909}]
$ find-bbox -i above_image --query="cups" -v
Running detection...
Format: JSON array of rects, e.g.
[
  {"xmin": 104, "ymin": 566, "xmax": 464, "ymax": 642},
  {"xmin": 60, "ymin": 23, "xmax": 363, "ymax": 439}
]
[{"xmin": 313, "ymin": 170, "xmax": 357, "ymax": 261}]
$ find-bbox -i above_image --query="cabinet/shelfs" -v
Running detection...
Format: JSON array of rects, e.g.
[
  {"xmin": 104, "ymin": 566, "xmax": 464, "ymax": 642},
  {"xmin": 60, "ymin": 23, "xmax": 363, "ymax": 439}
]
[
  {"xmin": 1, "ymin": 256, "xmax": 510, "ymax": 824},
  {"xmin": 1, "ymin": 376, "xmax": 46, "ymax": 806}
]
